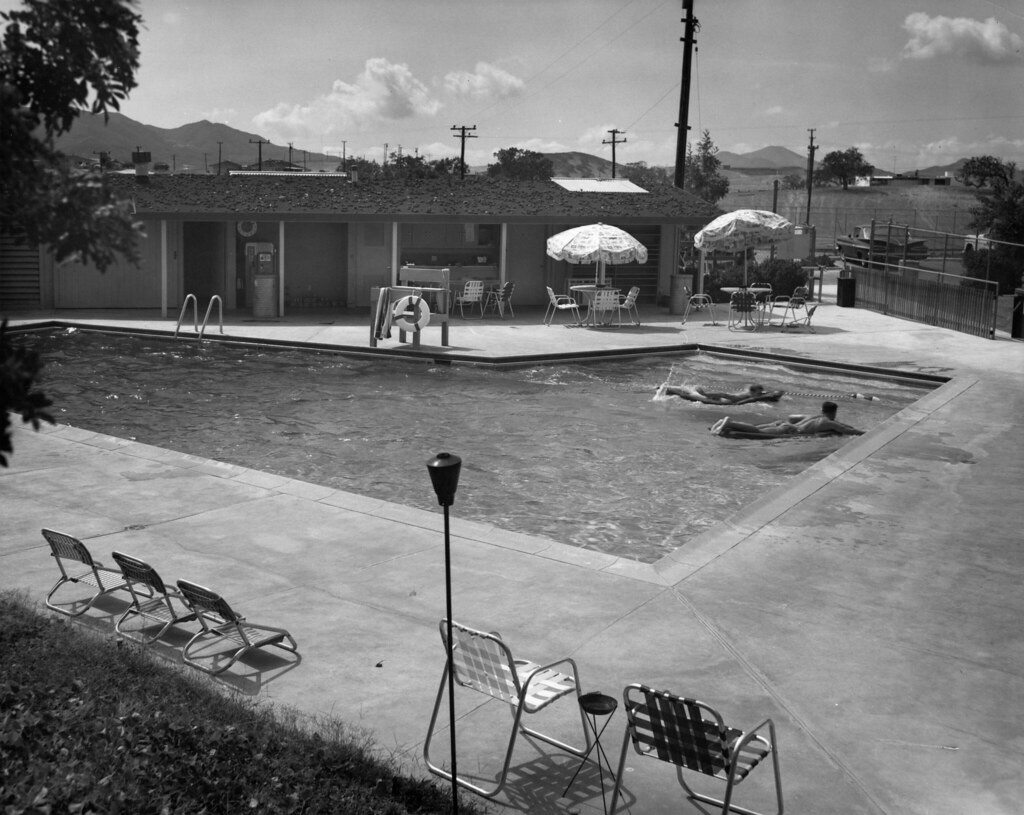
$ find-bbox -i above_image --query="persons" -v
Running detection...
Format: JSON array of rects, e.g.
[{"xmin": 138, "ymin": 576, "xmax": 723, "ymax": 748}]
[
  {"xmin": 709, "ymin": 402, "xmax": 866, "ymax": 438},
  {"xmin": 660, "ymin": 382, "xmax": 783, "ymax": 412}
]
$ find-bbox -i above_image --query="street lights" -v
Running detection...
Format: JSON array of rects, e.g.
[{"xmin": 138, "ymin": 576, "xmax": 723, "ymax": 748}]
[{"xmin": 422, "ymin": 450, "xmax": 465, "ymax": 804}]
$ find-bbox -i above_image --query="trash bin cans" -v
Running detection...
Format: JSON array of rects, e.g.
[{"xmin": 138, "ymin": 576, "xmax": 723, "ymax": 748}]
[
  {"xmin": 836, "ymin": 264, "xmax": 857, "ymax": 307},
  {"xmin": 669, "ymin": 271, "xmax": 694, "ymax": 316},
  {"xmin": 251, "ymin": 274, "xmax": 279, "ymax": 319}
]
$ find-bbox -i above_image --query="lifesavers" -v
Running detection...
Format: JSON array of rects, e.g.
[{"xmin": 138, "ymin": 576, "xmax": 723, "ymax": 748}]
[{"xmin": 391, "ymin": 293, "xmax": 433, "ymax": 334}]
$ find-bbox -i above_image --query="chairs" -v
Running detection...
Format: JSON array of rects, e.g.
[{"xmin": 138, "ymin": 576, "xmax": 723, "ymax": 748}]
[
  {"xmin": 423, "ymin": 619, "xmax": 590, "ymax": 798},
  {"xmin": 481, "ymin": 280, "xmax": 516, "ymax": 319},
  {"xmin": 608, "ymin": 286, "xmax": 640, "ymax": 328},
  {"xmin": 590, "ymin": 288, "xmax": 621, "ymax": 329},
  {"xmin": 749, "ymin": 283, "xmax": 772, "ymax": 327},
  {"xmin": 543, "ymin": 286, "xmax": 583, "ymax": 327},
  {"xmin": 609, "ymin": 683, "xmax": 785, "ymax": 815},
  {"xmin": 176, "ymin": 578, "xmax": 297, "ymax": 675},
  {"xmin": 112, "ymin": 551, "xmax": 228, "ymax": 644},
  {"xmin": 768, "ymin": 287, "xmax": 809, "ymax": 327},
  {"xmin": 728, "ymin": 292, "xmax": 758, "ymax": 332},
  {"xmin": 781, "ymin": 305, "xmax": 819, "ymax": 333},
  {"xmin": 452, "ymin": 281, "xmax": 485, "ymax": 319},
  {"xmin": 41, "ymin": 528, "xmax": 154, "ymax": 616},
  {"xmin": 681, "ymin": 285, "xmax": 718, "ymax": 326}
]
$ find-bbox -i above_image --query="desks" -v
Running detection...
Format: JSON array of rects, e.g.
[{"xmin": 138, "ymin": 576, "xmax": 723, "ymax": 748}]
[
  {"xmin": 721, "ymin": 287, "xmax": 771, "ymax": 330},
  {"xmin": 570, "ymin": 285, "xmax": 622, "ymax": 329}
]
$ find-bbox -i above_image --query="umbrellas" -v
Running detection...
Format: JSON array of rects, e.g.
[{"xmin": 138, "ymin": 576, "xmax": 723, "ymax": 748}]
[
  {"xmin": 695, "ymin": 208, "xmax": 795, "ymax": 331},
  {"xmin": 545, "ymin": 221, "xmax": 648, "ymax": 328}
]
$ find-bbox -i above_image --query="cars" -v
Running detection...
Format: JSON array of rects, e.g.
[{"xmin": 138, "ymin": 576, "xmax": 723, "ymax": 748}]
[
  {"xmin": 963, "ymin": 231, "xmax": 998, "ymax": 253},
  {"xmin": 833, "ymin": 223, "xmax": 930, "ymax": 270}
]
